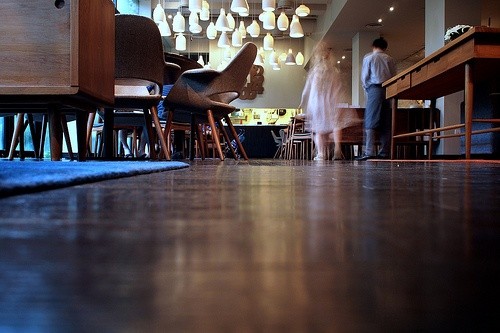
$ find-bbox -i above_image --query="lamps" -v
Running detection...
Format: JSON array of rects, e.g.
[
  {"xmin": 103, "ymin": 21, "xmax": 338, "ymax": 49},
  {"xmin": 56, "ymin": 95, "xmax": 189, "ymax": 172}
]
[{"xmin": 152, "ymin": 0, "xmax": 310, "ymax": 72}]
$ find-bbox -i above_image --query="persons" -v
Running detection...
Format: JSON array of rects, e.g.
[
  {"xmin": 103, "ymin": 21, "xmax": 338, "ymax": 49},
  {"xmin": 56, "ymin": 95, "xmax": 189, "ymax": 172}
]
[
  {"xmin": 354, "ymin": 37, "xmax": 396, "ymax": 161},
  {"xmin": 298, "ymin": 41, "xmax": 358, "ymax": 161}
]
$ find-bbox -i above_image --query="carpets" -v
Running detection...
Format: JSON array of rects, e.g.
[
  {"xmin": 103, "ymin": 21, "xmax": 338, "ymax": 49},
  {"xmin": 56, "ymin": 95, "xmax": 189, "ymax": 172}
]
[{"xmin": 0, "ymin": 158, "xmax": 191, "ymax": 198}]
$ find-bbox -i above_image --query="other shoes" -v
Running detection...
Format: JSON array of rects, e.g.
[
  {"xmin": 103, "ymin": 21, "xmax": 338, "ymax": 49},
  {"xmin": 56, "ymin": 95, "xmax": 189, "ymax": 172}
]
[
  {"xmin": 353, "ymin": 155, "xmax": 377, "ymax": 161},
  {"xmin": 331, "ymin": 155, "xmax": 344, "ymax": 161},
  {"xmin": 313, "ymin": 154, "xmax": 328, "ymax": 161}
]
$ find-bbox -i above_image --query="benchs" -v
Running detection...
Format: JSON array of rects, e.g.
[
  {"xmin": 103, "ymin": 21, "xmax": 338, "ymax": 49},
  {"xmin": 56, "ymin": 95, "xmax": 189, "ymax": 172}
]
[{"xmin": 323, "ymin": 107, "xmax": 441, "ymax": 163}]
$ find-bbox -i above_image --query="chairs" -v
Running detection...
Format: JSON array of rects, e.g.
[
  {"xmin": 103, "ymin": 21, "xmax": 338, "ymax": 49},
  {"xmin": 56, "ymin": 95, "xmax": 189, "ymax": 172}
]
[{"xmin": 114, "ymin": 14, "xmax": 259, "ymax": 166}]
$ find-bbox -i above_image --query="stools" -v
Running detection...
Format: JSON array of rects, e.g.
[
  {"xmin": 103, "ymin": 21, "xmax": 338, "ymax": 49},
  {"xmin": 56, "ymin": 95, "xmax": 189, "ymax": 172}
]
[{"xmin": 271, "ymin": 116, "xmax": 313, "ymax": 160}]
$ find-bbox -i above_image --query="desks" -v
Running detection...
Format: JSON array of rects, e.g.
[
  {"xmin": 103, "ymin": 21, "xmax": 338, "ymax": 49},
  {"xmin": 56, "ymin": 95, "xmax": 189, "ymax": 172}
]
[
  {"xmin": 0, "ymin": 0, "xmax": 115, "ymax": 161},
  {"xmin": 382, "ymin": 27, "xmax": 500, "ymax": 161}
]
